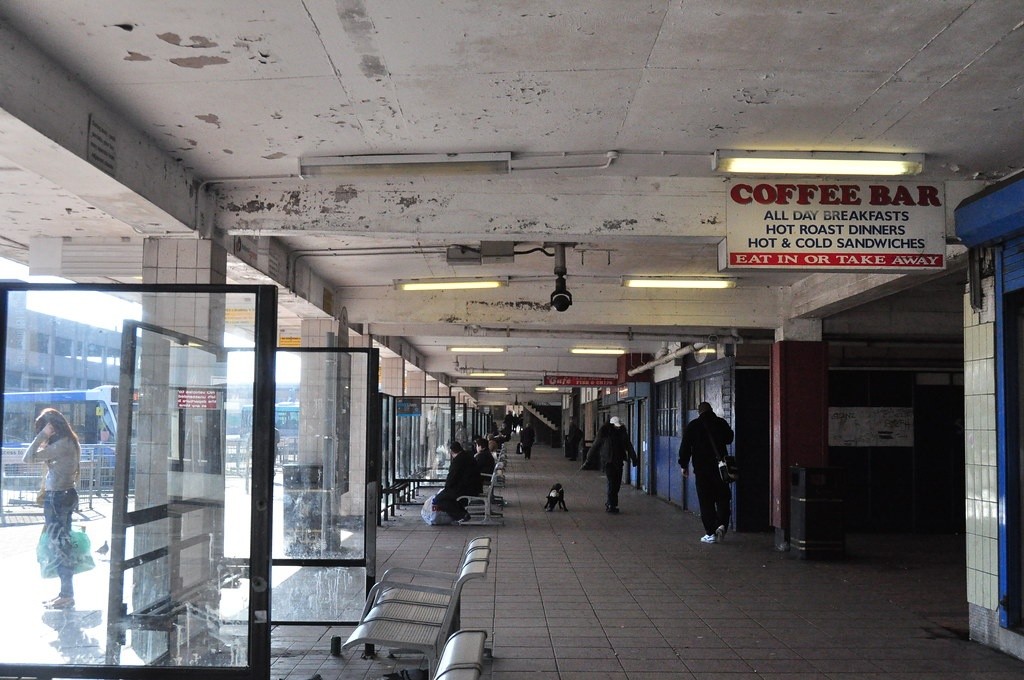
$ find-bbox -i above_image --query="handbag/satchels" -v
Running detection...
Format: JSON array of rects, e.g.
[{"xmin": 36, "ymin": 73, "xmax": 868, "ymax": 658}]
[
  {"xmin": 516, "ymin": 443, "xmax": 523, "ymax": 454},
  {"xmin": 37, "ymin": 523, "xmax": 96, "ymax": 578},
  {"xmin": 718, "ymin": 457, "xmax": 739, "ymax": 484}
]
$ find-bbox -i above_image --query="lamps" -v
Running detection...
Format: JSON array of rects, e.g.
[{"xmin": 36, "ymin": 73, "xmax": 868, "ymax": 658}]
[
  {"xmin": 467, "ymin": 371, "xmax": 508, "ymax": 378},
  {"xmin": 621, "ymin": 276, "xmax": 740, "ymax": 290},
  {"xmin": 568, "ymin": 348, "xmax": 629, "ymax": 355},
  {"xmin": 392, "ymin": 276, "xmax": 509, "ymax": 292},
  {"xmin": 710, "ymin": 147, "xmax": 925, "ymax": 178},
  {"xmin": 295, "ymin": 149, "xmax": 514, "ymax": 182},
  {"xmin": 445, "ymin": 346, "xmax": 509, "ymax": 353}
]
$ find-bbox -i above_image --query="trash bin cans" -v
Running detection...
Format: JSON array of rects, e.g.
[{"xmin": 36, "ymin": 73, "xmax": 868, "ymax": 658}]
[
  {"xmin": 787, "ymin": 465, "xmax": 846, "ymax": 562},
  {"xmin": 582, "ymin": 440, "xmax": 601, "ymax": 470},
  {"xmin": 282, "ymin": 464, "xmax": 340, "ymax": 552},
  {"xmin": 565, "ymin": 435, "xmax": 578, "ymax": 461}
]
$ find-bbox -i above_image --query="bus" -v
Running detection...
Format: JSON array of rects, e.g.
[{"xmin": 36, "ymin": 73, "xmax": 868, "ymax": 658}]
[
  {"xmin": 1, "ymin": 384, "xmax": 138, "ymax": 491},
  {"xmin": 276, "ymin": 401, "xmax": 302, "ymax": 454},
  {"xmin": 224, "ymin": 402, "xmax": 252, "ymax": 463}
]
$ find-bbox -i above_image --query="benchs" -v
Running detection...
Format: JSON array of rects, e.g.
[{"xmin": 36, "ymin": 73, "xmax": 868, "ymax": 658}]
[
  {"xmin": 341, "ymin": 537, "xmax": 493, "ymax": 680},
  {"xmin": 381, "ymin": 479, "xmax": 409, "ymax": 497},
  {"xmin": 456, "ymin": 440, "xmax": 508, "ymax": 528},
  {"xmin": 407, "ymin": 469, "xmax": 429, "ymax": 479}
]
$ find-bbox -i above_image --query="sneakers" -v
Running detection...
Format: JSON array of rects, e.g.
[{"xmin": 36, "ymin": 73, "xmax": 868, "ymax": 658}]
[
  {"xmin": 715, "ymin": 525, "xmax": 725, "ymax": 543},
  {"xmin": 451, "ymin": 508, "xmax": 471, "ymax": 526},
  {"xmin": 700, "ymin": 534, "xmax": 716, "ymax": 543},
  {"xmin": 608, "ymin": 505, "xmax": 619, "ymax": 513}
]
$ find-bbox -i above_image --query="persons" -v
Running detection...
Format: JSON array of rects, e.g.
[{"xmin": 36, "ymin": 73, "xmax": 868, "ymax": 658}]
[
  {"xmin": 569, "ymin": 423, "xmax": 581, "ymax": 461},
  {"xmin": 23, "ymin": 408, "xmax": 81, "ymax": 609},
  {"xmin": 678, "ymin": 402, "xmax": 734, "ymax": 544},
  {"xmin": 505, "ymin": 411, "xmax": 524, "ymax": 431},
  {"xmin": 586, "ymin": 416, "xmax": 637, "ymax": 513},
  {"xmin": 487, "ymin": 434, "xmax": 497, "ymax": 452},
  {"xmin": 493, "ymin": 432, "xmax": 502, "ymax": 449},
  {"xmin": 474, "ymin": 439, "xmax": 495, "ymax": 484},
  {"xmin": 521, "ymin": 423, "xmax": 535, "ymax": 459},
  {"xmin": 433, "ymin": 442, "xmax": 483, "ymax": 526}
]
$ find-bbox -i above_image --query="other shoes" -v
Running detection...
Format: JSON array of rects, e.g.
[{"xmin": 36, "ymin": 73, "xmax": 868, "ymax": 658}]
[
  {"xmin": 43, "ymin": 595, "xmax": 59, "ymax": 605},
  {"xmin": 44, "ymin": 598, "xmax": 75, "ymax": 611}
]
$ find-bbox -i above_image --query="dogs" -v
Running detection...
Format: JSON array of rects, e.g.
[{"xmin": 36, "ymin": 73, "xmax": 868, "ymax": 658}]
[{"xmin": 544, "ymin": 483, "xmax": 568, "ymax": 512}]
